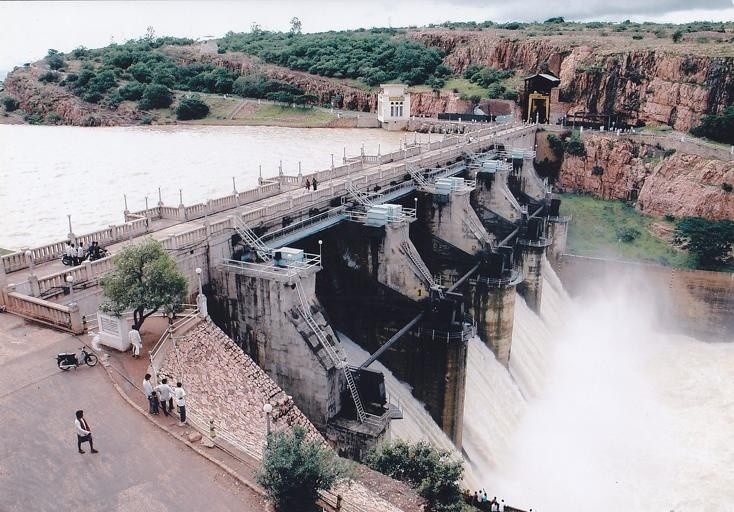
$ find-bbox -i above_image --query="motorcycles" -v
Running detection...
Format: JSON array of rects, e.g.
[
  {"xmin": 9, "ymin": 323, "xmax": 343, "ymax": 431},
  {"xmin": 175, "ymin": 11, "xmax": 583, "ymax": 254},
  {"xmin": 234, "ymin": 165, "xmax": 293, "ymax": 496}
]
[
  {"xmin": 54, "ymin": 345, "xmax": 97, "ymax": 372},
  {"xmin": 62, "ymin": 244, "xmax": 109, "ymax": 265}
]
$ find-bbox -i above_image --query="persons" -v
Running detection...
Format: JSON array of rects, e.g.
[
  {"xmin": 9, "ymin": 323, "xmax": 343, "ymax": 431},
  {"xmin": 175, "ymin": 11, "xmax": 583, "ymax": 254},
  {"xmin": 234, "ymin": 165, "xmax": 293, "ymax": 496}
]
[
  {"xmin": 143, "ymin": 374, "xmax": 186, "ymax": 423},
  {"xmin": 306, "ymin": 178, "xmax": 317, "ymax": 190},
  {"xmin": 66, "ymin": 240, "xmax": 105, "ymax": 266},
  {"xmin": 166, "ymin": 303, "xmax": 174, "ymax": 325},
  {"xmin": 128, "ymin": 325, "xmax": 141, "ymax": 357},
  {"xmin": 75, "ymin": 410, "xmax": 98, "ymax": 453}
]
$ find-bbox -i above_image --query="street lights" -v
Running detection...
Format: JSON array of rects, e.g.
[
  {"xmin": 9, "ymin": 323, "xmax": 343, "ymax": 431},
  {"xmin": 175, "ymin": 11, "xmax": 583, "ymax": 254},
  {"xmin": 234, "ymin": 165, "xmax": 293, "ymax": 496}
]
[
  {"xmin": 378, "ymin": 155, "xmax": 381, "ymax": 170},
  {"xmin": 235, "ymin": 193, "xmax": 241, "ymax": 216},
  {"xmin": 474, "ymin": 172, "xmax": 477, "ymax": 189},
  {"xmin": 414, "ymin": 131, "xmax": 417, "ymax": 143},
  {"xmin": 66, "ymin": 209, "xmax": 73, "ymax": 233},
  {"xmin": 443, "ymin": 129, "xmax": 446, "ymax": 140},
  {"xmin": 202, "ymin": 201, "xmax": 207, "ymax": 226},
  {"xmin": 179, "ymin": 186, "xmax": 183, "ymax": 206},
  {"xmin": 428, "ymin": 131, "xmax": 432, "ymax": 143},
  {"xmin": 144, "ymin": 195, "xmax": 149, "ymax": 211},
  {"xmin": 399, "ymin": 137, "xmax": 403, "ymax": 150},
  {"xmin": 67, "ymin": 275, "xmax": 76, "ymax": 304},
  {"xmin": 123, "ymin": 194, "xmax": 128, "ymax": 208},
  {"xmin": 157, "ymin": 186, "xmax": 161, "ymax": 202},
  {"xmin": 232, "ymin": 176, "xmax": 237, "ymax": 193},
  {"xmin": 404, "ymin": 131, "xmax": 407, "ymax": 146},
  {"xmin": 478, "ymin": 130, "xmax": 481, "ymax": 142},
  {"xmin": 361, "ymin": 142, "xmax": 365, "ymax": 156},
  {"xmin": 456, "ymin": 136, "xmax": 459, "ymax": 147},
  {"xmin": 298, "ymin": 161, "xmax": 302, "ymax": 176},
  {"xmin": 195, "ymin": 266, "xmax": 203, "ymax": 296},
  {"xmin": 318, "ymin": 240, "xmax": 322, "ymax": 266},
  {"xmin": 523, "ymin": 120, "xmax": 525, "ymax": 128},
  {"xmin": 343, "ymin": 145, "xmax": 346, "ymax": 158},
  {"xmin": 330, "ymin": 153, "xmax": 333, "ymax": 168},
  {"xmin": 505, "ymin": 124, "xmax": 508, "ymax": 134},
  {"xmin": 263, "ymin": 404, "xmax": 276, "ymax": 442},
  {"xmin": 288, "ymin": 179, "xmax": 292, "ymax": 199},
  {"xmin": 24, "ymin": 249, "xmax": 34, "ymax": 277},
  {"xmin": 258, "ymin": 165, "xmax": 263, "ymax": 180},
  {"xmin": 414, "ymin": 198, "xmax": 419, "ymax": 219},
  {"xmin": 404, "ymin": 149, "xmax": 408, "ymax": 160},
  {"xmin": 278, "ymin": 160, "xmax": 283, "ymax": 176},
  {"xmin": 345, "ymin": 164, "xmax": 351, "ymax": 178},
  {"xmin": 489, "ymin": 127, "xmax": 493, "ymax": 139}
]
